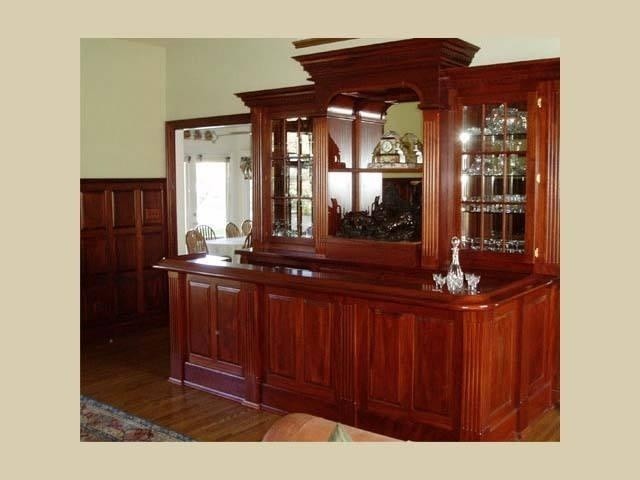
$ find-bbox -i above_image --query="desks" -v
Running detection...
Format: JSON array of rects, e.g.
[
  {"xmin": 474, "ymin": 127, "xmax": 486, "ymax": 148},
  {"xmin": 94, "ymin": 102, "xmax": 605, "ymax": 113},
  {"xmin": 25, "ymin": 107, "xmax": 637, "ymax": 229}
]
[{"xmin": 203, "ymin": 236, "xmax": 251, "ymax": 264}]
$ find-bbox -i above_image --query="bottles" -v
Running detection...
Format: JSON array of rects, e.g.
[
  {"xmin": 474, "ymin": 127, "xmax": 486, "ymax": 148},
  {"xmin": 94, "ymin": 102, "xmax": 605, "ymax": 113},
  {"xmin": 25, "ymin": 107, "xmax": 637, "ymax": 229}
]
[{"xmin": 446, "ymin": 235, "xmax": 465, "ymax": 296}]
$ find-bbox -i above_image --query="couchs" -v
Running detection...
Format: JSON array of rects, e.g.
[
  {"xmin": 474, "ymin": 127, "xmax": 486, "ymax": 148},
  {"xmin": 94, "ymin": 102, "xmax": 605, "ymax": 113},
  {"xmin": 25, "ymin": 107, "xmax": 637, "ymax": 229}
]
[{"xmin": 263, "ymin": 412, "xmax": 415, "ymax": 442}]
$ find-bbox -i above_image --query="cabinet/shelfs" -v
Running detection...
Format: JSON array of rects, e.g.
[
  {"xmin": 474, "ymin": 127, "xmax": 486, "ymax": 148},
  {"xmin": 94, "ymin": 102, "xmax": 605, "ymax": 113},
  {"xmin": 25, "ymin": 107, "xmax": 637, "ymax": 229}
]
[
  {"xmin": 288, "ymin": 38, "xmax": 481, "ymax": 269},
  {"xmin": 235, "ymin": 82, "xmax": 317, "ymax": 252},
  {"xmin": 439, "ymin": 58, "xmax": 561, "ymax": 275}
]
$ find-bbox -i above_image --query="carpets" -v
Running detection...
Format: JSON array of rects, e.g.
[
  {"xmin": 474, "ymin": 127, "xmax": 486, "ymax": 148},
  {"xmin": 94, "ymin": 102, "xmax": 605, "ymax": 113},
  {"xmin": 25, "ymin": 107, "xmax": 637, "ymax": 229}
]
[{"xmin": 80, "ymin": 394, "xmax": 195, "ymax": 442}]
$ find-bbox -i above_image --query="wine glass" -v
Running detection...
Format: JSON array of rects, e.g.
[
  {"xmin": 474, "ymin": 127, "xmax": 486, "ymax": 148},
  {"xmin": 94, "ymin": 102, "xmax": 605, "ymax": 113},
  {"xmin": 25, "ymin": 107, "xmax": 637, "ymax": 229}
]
[
  {"xmin": 466, "ymin": 273, "xmax": 481, "ymax": 295},
  {"xmin": 432, "ymin": 274, "xmax": 447, "ymax": 294},
  {"xmin": 468, "ymin": 194, "xmax": 527, "ymax": 213}
]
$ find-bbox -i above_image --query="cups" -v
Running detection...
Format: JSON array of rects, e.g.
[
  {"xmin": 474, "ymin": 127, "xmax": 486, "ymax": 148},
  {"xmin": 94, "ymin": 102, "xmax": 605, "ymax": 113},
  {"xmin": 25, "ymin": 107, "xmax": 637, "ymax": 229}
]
[{"xmin": 462, "ymin": 237, "xmax": 525, "ymax": 255}]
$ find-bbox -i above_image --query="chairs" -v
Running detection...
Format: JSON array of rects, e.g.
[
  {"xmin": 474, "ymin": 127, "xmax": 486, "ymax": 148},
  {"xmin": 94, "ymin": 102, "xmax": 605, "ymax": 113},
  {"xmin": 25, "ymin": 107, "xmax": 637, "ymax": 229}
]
[
  {"xmin": 185, "ymin": 229, "xmax": 209, "ymax": 255},
  {"xmin": 226, "ymin": 222, "xmax": 240, "ymax": 237},
  {"xmin": 241, "ymin": 219, "xmax": 253, "ymax": 237},
  {"xmin": 196, "ymin": 224, "xmax": 217, "ymax": 240}
]
